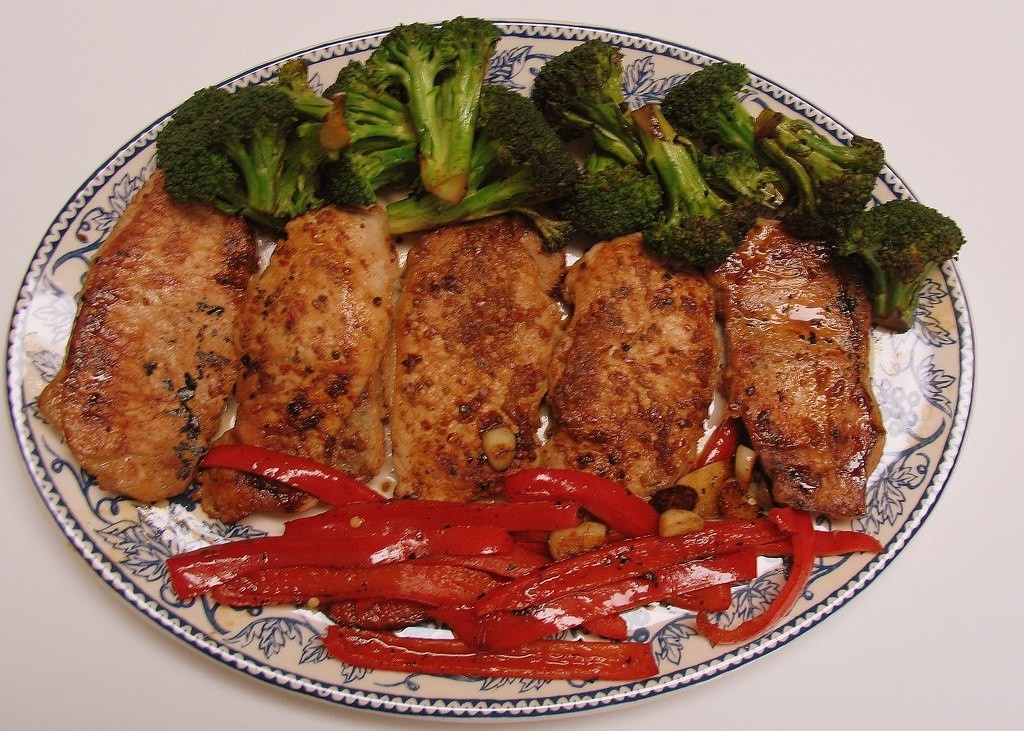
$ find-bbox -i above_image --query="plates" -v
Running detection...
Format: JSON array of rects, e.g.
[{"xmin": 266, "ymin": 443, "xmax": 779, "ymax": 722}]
[{"xmin": 4, "ymin": 19, "xmax": 977, "ymax": 725}]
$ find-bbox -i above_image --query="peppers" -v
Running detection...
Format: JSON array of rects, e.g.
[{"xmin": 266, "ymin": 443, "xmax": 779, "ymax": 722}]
[{"xmin": 165, "ymin": 419, "xmax": 885, "ymax": 681}]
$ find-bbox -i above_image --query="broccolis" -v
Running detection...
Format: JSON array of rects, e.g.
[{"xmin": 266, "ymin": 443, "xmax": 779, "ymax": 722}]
[{"xmin": 155, "ymin": 16, "xmax": 967, "ymax": 332}]
